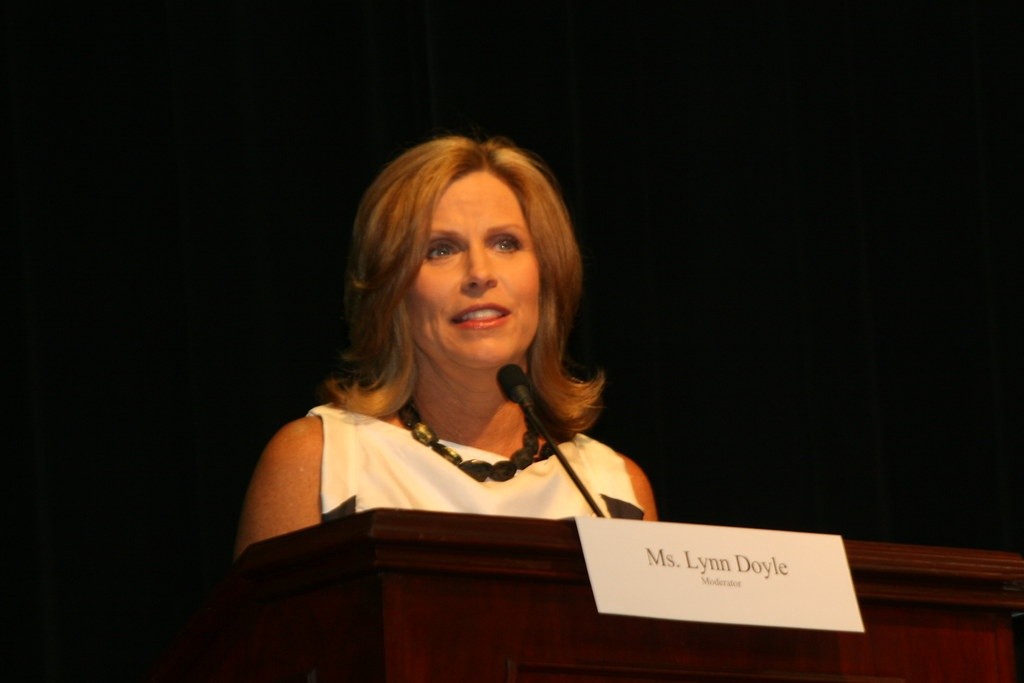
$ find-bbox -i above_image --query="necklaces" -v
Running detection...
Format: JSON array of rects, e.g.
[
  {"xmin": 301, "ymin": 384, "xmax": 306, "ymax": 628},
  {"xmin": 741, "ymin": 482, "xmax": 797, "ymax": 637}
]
[{"xmin": 394, "ymin": 399, "xmax": 540, "ymax": 482}]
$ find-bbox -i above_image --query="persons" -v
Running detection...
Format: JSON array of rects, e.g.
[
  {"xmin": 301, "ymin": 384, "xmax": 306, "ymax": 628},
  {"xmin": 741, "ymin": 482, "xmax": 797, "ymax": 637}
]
[{"xmin": 233, "ymin": 137, "xmax": 658, "ymax": 561}]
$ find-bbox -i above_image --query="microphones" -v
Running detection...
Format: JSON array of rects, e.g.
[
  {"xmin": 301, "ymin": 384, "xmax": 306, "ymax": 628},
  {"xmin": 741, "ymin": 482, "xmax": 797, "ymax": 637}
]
[{"xmin": 496, "ymin": 364, "xmax": 604, "ymax": 518}]
